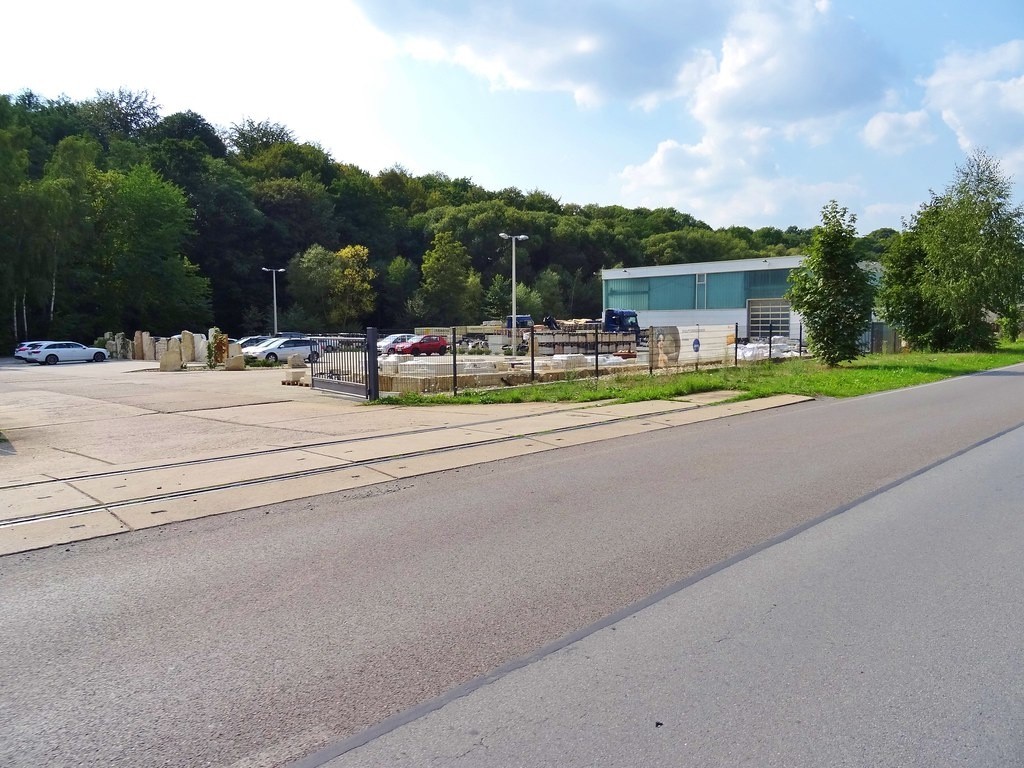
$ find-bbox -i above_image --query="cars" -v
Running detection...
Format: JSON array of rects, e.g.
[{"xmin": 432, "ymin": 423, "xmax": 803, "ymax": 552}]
[
  {"xmin": 395, "ymin": 335, "xmax": 447, "ymax": 356},
  {"xmin": 448, "ymin": 338, "xmax": 488, "ymax": 353},
  {"xmin": 320, "ymin": 338, "xmax": 339, "ymax": 352},
  {"xmin": 377, "ymin": 334, "xmax": 418, "ymax": 356},
  {"xmin": 235, "ymin": 336, "xmax": 325, "ymax": 362},
  {"xmin": 15, "ymin": 342, "xmax": 54, "ymax": 363},
  {"xmin": 27, "ymin": 341, "xmax": 110, "ymax": 365}
]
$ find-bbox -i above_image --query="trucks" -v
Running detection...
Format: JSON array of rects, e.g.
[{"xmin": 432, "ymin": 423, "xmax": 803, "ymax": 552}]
[
  {"xmin": 544, "ymin": 309, "xmax": 641, "ymax": 346},
  {"xmin": 450, "ymin": 315, "xmax": 534, "ymax": 340}
]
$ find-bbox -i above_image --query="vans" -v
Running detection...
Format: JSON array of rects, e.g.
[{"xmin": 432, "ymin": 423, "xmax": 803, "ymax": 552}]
[{"xmin": 275, "ymin": 332, "xmax": 306, "ymax": 343}]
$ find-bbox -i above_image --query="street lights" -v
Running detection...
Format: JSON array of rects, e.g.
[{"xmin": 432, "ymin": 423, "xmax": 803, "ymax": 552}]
[
  {"xmin": 499, "ymin": 233, "xmax": 529, "ymax": 355},
  {"xmin": 262, "ymin": 267, "xmax": 286, "ymax": 335}
]
